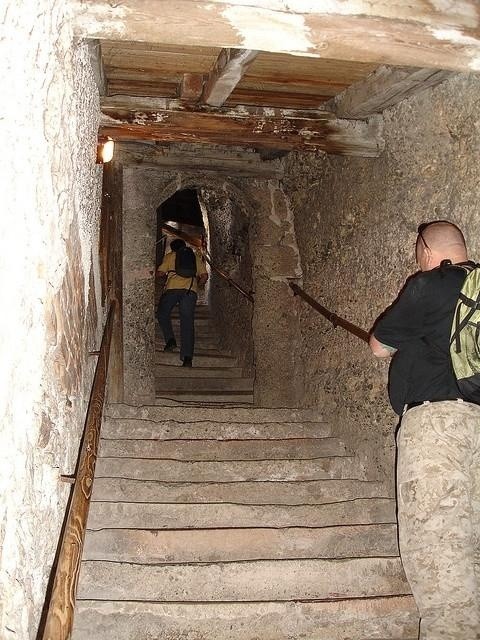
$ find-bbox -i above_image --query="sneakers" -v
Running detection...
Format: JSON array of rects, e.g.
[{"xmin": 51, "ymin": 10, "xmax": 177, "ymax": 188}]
[
  {"xmin": 181, "ymin": 356, "xmax": 193, "ymax": 367},
  {"xmin": 163, "ymin": 339, "xmax": 177, "ymax": 351}
]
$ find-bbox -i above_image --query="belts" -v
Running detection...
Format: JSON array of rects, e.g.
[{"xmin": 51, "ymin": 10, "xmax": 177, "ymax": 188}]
[{"xmin": 405, "ymin": 398, "xmax": 480, "ymax": 411}]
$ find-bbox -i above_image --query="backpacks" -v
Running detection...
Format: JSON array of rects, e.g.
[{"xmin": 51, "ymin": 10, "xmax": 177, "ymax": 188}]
[
  {"xmin": 431, "ymin": 262, "xmax": 480, "ymax": 402},
  {"xmin": 167, "ymin": 246, "xmax": 199, "ymax": 278}
]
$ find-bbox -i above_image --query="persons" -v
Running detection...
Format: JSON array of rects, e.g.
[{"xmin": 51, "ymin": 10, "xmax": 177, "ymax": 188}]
[
  {"xmin": 155, "ymin": 238, "xmax": 209, "ymax": 369},
  {"xmin": 368, "ymin": 219, "xmax": 480, "ymax": 638}
]
